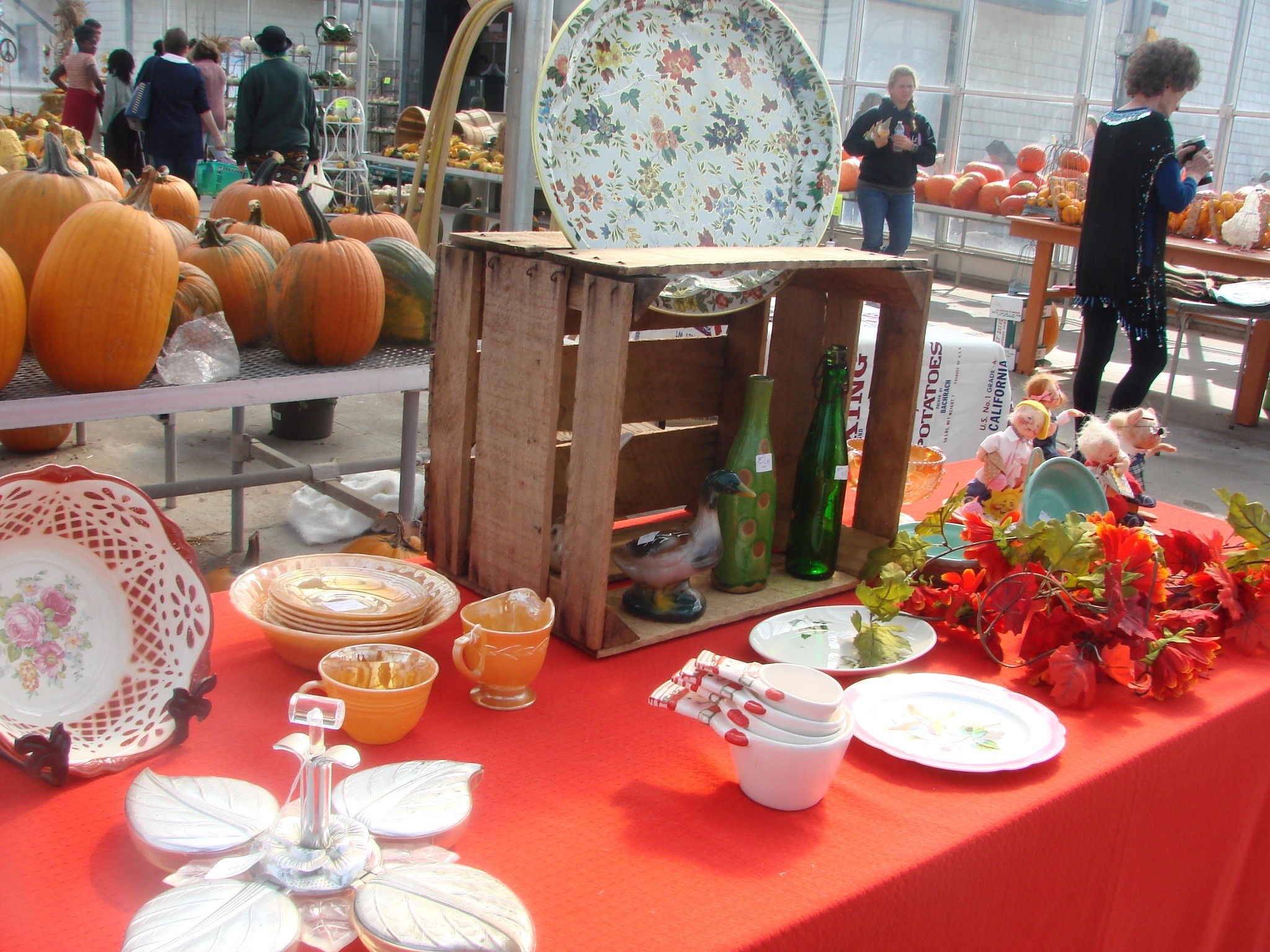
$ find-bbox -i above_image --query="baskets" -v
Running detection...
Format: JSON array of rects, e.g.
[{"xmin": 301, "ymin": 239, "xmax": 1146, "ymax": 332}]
[{"xmin": 195, "ymin": 145, "xmax": 251, "ymax": 198}]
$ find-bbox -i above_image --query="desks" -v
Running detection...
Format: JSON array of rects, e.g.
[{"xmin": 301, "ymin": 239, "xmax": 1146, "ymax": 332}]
[
  {"xmin": 359, "ymin": 150, "xmax": 543, "ymax": 235},
  {"xmin": 1, "ymin": 338, "xmax": 433, "ymax": 564},
  {"xmin": 910, "ymin": 201, "xmax": 1073, "ymax": 323},
  {"xmin": 0, "ymin": 445, "xmax": 1270, "ymax": 950},
  {"xmin": 1004, "ymin": 213, "xmax": 1270, "ymax": 428}
]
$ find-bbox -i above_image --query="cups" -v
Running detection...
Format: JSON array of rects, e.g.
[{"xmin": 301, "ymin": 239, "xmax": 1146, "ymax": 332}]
[
  {"xmin": 453, "ymin": 586, "xmax": 557, "ymax": 710},
  {"xmin": 298, "ymin": 643, "xmax": 440, "ymax": 746}
]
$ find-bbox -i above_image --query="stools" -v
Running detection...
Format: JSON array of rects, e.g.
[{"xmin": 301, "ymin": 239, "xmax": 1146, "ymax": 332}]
[{"xmin": 1167, "ymin": 294, "xmax": 1270, "ymax": 431}]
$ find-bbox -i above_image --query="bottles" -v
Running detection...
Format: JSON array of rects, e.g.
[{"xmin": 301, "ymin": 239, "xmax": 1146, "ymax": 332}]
[
  {"xmin": 715, "ymin": 373, "xmax": 780, "ymax": 595},
  {"xmin": 786, "ymin": 344, "xmax": 850, "ymax": 581},
  {"xmin": 892, "ymin": 121, "xmax": 906, "ymax": 152}
]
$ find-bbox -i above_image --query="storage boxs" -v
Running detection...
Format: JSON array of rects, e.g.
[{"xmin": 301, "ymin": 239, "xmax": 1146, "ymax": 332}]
[
  {"xmin": 992, "ymin": 318, "xmax": 1047, "ymax": 373},
  {"xmin": 988, "ymin": 293, "xmax": 1054, "ymax": 323}
]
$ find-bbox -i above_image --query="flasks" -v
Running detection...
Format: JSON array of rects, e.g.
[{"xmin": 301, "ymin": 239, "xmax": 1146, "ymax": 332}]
[{"xmin": 1182, "ymin": 135, "xmax": 1214, "ymax": 187}]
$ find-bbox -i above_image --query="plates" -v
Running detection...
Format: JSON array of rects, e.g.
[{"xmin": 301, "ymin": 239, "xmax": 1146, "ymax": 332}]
[
  {"xmin": 842, "ymin": 673, "xmax": 1068, "ymax": 771},
  {"xmin": 264, "ymin": 567, "xmax": 429, "ymax": 632},
  {"xmin": 747, "ymin": 603, "xmax": 938, "ymax": 676},
  {"xmin": 529, "ymin": 0, "xmax": 845, "ymax": 319}
]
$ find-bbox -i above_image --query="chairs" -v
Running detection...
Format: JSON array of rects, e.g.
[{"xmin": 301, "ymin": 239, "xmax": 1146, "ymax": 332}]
[{"xmin": 315, "ymin": 95, "xmax": 370, "ymax": 213}]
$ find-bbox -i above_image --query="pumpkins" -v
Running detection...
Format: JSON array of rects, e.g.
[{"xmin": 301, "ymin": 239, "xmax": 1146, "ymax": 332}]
[
  {"xmin": 1042, "ymin": 302, "xmax": 1059, "ymax": 354},
  {"xmin": 2, "ymin": 108, "xmax": 543, "ymax": 393},
  {"xmin": 838, "ymin": 145, "xmax": 1270, "ymax": 251}
]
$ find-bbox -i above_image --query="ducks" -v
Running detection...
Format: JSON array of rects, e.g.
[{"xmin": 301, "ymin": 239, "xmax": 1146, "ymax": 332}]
[{"xmin": 610, "ymin": 468, "xmax": 757, "ymax": 623}]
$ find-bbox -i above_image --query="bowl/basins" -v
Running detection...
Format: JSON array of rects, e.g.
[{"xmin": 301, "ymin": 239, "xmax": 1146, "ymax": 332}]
[
  {"xmin": 0, "ymin": 460, "xmax": 216, "ymax": 783},
  {"xmin": 899, "ymin": 520, "xmax": 980, "ymax": 584},
  {"xmin": 1024, "ymin": 444, "xmax": 1107, "ymax": 533},
  {"xmin": 645, "ymin": 647, "xmax": 856, "ymax": 810},
  {"xmin": 845, "ymin": 437, "xmax": 944, "ymax": 506},
  {"xmin": 227, "ymin": 550, "xmax": 461, "ymax": 672}
]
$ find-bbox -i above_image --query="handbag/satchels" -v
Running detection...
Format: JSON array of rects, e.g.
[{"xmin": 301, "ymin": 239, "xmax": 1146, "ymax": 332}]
[
  {"xmin": 301, "ymin": 160, "xmax": 333, "ymax": 214},
  {"xmin": 125, "ymin": 55, "xmax": 157, "ymax": 134}
]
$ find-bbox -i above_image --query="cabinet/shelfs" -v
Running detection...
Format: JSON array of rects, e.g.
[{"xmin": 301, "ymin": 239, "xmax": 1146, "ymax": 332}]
[
  {"xmin": 368, "ymin": 71, "xmax": 400, "ymax": 152},
  {"xmin": 310, "ymin": 41, "xmax": 358, "ymax": 173}
]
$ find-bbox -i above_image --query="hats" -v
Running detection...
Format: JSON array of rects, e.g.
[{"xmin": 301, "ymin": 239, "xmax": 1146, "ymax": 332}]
[{"xmin": 254, "ymin": 26, "xmax": 292, "ymax": 52}]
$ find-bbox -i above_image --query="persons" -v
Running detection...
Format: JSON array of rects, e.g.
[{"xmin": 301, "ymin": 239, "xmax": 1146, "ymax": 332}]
[
  {"xmin": 49, "ymin": 18, "xmax": 109, "ymax": 156},
  {"xmin": 851, "ymin": 92, "xmax": 883, "ymax": 127},
  {"xmin": 235, "ymin": 26, "xmax": 321, "ymax": 188},
  {"xmin": 1081, "ymin": 116, "xmax": 1098, "ymax": 174},
  {"xmin": 961, "ymin": 370, "xmax": 1177, "ymax": 529},
  {"xmin": 187, "ymin": 39, "xmax": 226, "ymax": 161},
  {"xmin": 133, "ymin": 40, "xmax": 164, "ymax": 88},
  {"xmin": 841, "ymin": 65, "xmax": 936, "ymax": 257},
  {"xmin": 102, "ymin": 48, "xmax": 145, "ymax": 180},
  {"xmin": 985, "ymin": 140, "xmax": 1017, "ymax": 165},
  {"xmin": 1068, "ymin": 38, "xmax": 1216, "ymax": 507},
  {"xmin": 138, "ymin": 27, "xmax": 225, "ymax": 186}
]
